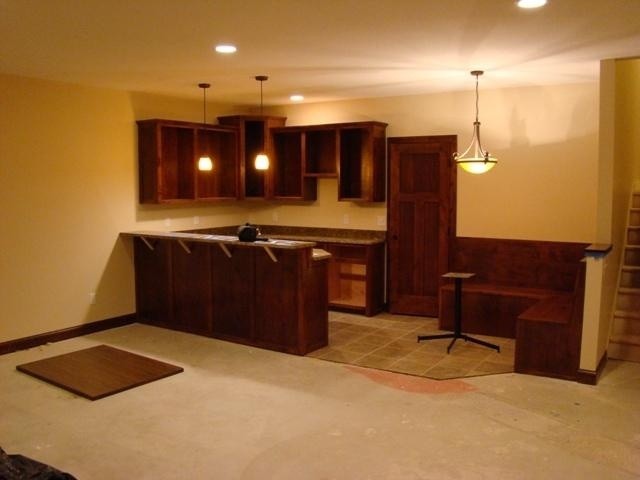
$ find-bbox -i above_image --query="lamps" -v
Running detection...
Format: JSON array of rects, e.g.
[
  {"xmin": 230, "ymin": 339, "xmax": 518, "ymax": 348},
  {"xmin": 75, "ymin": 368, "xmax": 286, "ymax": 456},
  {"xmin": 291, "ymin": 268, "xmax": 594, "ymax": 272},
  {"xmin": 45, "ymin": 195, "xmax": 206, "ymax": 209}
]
[
  {"xmin": 452, "ymin": 70, "xmax": 497, "ymax": 174},
  {"xmin": 255, "ymin": 76, "xmax": 269, "ymax": 169},
  {"xmin": 198, "ymin": 83, "xmax": 212, "ymax": 171}
]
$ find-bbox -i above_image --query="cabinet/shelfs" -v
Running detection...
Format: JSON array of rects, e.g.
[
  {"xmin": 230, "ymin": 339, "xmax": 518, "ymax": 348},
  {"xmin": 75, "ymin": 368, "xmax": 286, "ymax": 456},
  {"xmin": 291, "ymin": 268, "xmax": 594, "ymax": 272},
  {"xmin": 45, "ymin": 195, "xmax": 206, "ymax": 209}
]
[
  {"xmin": 135, "ymin": 115, "xmax": 389, "ymax": 205},
  {"xmin": 326, "ymin": 242, "xmax": 384, "ymax": 317}
]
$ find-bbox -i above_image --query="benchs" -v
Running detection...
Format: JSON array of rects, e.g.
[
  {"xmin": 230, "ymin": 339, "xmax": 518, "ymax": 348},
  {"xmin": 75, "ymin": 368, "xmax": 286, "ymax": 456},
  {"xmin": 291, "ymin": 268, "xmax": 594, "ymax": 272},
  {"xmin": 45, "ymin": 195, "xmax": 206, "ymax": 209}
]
[{"xmin": 439, "ymin": 236, "xmax": 592, "ymax": 380}]
